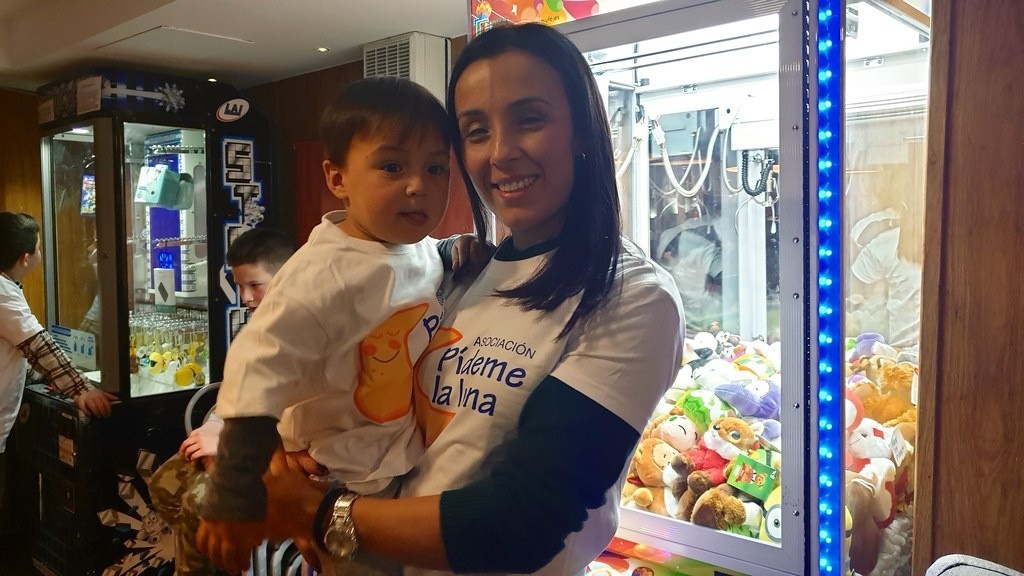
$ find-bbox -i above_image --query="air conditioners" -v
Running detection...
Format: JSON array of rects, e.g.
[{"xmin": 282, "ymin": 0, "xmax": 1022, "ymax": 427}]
[{"xmin": 363, "ymin": 31, "xmax": 453, "ymax": 114}]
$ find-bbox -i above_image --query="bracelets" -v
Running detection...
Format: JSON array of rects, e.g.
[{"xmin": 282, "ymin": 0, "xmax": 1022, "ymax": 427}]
[{"xmin": 310, "ymin": 486, "xmax": 345, "ymax": 556}]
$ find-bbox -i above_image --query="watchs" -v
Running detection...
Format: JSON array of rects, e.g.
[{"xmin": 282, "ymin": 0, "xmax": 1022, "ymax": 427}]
[{"xmin": 322, "ymin": 490, "xmax": 360, "ymax": 562}]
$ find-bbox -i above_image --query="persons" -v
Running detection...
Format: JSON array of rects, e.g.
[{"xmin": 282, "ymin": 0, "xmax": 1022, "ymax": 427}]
[
  {"xmin": 178, "ymin": 227, "xmax": 299, "ymax": 463},
  {"xmin": 0, "ymin": 210, "xmax": 120, "ymax": 575},
  {"xmin": 70, "ymin": 224, "xmax": 101, "ymax": 372},
  {"xmin": 195, "ymin": 21, "xmax": 688, "ymax": 576}
]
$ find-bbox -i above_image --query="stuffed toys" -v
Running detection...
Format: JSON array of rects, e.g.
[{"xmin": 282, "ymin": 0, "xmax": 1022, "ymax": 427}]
[{"xmin": 622, "ymin": 328, "xmax": 919, "ymax": 576}]
